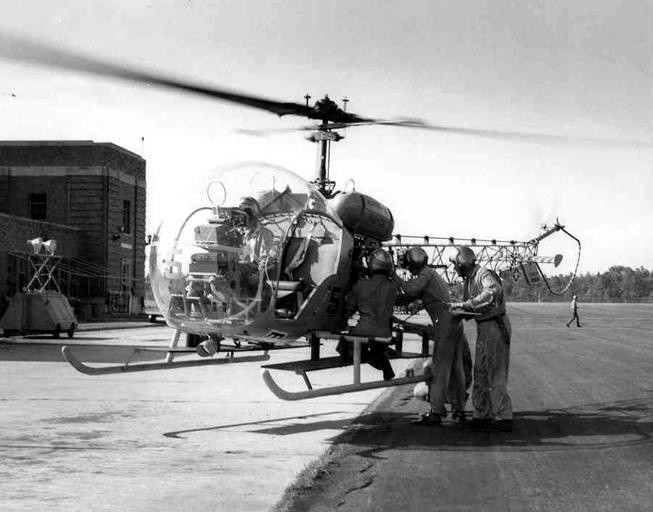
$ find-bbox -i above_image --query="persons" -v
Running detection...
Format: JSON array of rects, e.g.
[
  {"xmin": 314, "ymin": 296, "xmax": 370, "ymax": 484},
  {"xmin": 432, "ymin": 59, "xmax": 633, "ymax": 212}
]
[
  {"xmin": 441, "ymin": 245, "xmax": 516, "ymax": 432},
  {"xmin": 565, "ymin": 294, "xmax": 581, "ymax": 328},
  {"xmin": 335, "ymin": 246, "xmax": 397, "ymax": 383},
  {"xmin": 184, "ymin": 191, "xmax": 276, "ymax": 313},
  {"xmin": 388, "ymin": 243, "xmax": 472, "ymax": 428}
]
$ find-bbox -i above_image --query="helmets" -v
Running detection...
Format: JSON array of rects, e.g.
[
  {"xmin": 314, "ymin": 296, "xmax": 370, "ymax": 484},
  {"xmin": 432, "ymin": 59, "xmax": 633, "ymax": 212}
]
[
  {"xmin": 399, "ymin": 248, "xmax": 428, "ymax": 275},
  {"xmin": 449, "ymin": 245, "xmax": 477, "ymax": 276},
  {"xmin": 368, "ymin": 248, "xmax": 393, "ymax": 276}
]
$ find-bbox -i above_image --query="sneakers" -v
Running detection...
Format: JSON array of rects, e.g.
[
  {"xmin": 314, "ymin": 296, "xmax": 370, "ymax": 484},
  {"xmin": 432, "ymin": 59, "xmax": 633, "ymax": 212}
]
[
  {"xmin": 411, "ymin": 411, "xmax": 442, "ymax": 426},
  {"xmin": 452, "ymin": 409, "xmax": 466, "ymax": 421},
  {"xmin": 468, "ymin": 419, "xmax": 513, "ymax": 432}
]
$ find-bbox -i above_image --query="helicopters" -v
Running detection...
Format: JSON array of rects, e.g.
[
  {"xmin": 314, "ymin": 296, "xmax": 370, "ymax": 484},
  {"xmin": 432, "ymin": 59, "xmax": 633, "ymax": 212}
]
[{"xmin": 0, "ymin": 30, "xmax": 652, "ymax": 403}]
